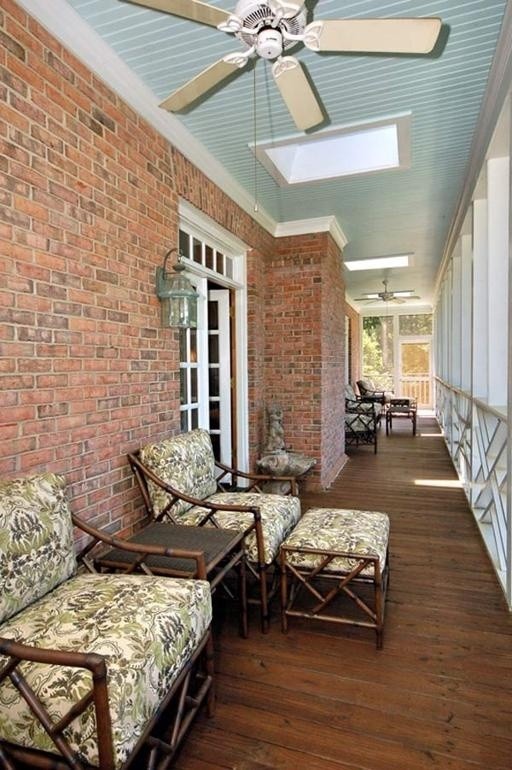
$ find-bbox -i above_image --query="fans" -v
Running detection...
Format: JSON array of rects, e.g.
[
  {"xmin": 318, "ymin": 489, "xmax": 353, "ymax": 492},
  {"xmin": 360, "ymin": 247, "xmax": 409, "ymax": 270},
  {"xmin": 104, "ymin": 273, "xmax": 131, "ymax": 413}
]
[
  {"xmin": 353, "ymin": 278, "xmax": 420, "ymax": 307},
  {"xmin": 119, "ymin": 0, "xmax": 444, "ymax": 134}
]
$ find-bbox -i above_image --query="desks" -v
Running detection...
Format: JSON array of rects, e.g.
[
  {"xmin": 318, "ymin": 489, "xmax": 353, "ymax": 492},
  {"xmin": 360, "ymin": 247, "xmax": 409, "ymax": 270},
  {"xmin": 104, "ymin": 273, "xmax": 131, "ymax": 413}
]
[{"xmin": 100, "ymin": 520, "xmax": 250, "ymax": 640}]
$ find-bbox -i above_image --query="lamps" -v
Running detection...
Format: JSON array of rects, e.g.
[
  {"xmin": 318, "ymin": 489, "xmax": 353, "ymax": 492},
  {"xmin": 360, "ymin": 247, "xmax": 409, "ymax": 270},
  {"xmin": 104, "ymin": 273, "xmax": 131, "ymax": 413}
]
[
  {"xmin": 253, "ymin": 28, "xmax": 284, "ymax": 61},
  {"xmin": 154, "ymin": 249, "xmax": 200, "ymax": 331}
]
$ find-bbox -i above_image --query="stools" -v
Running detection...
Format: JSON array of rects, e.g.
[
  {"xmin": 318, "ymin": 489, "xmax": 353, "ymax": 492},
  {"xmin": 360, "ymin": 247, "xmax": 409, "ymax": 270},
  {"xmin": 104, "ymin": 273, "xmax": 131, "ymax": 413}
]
[
  {"xmin": 386, "ymin": 398, "xmax": 418, "ymax": 437},
  {"xmin": 283, "ymin": 506, "xmax": 391, "ymax": 650}
]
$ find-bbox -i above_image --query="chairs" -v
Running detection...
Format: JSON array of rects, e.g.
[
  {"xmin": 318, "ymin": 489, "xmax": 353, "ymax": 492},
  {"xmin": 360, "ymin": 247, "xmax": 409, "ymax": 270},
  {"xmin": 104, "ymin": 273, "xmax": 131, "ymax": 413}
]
[
  {"xmin": 1, "ymin": 471, "xmax": 216, "ymax": 769},
  {"xmin": 126, "ymin": 430, "xmax": 302, "ymax": 635},
  {"xmin": 344, "ymin": 380, "xmax": 394, "ymax": 454}
]
